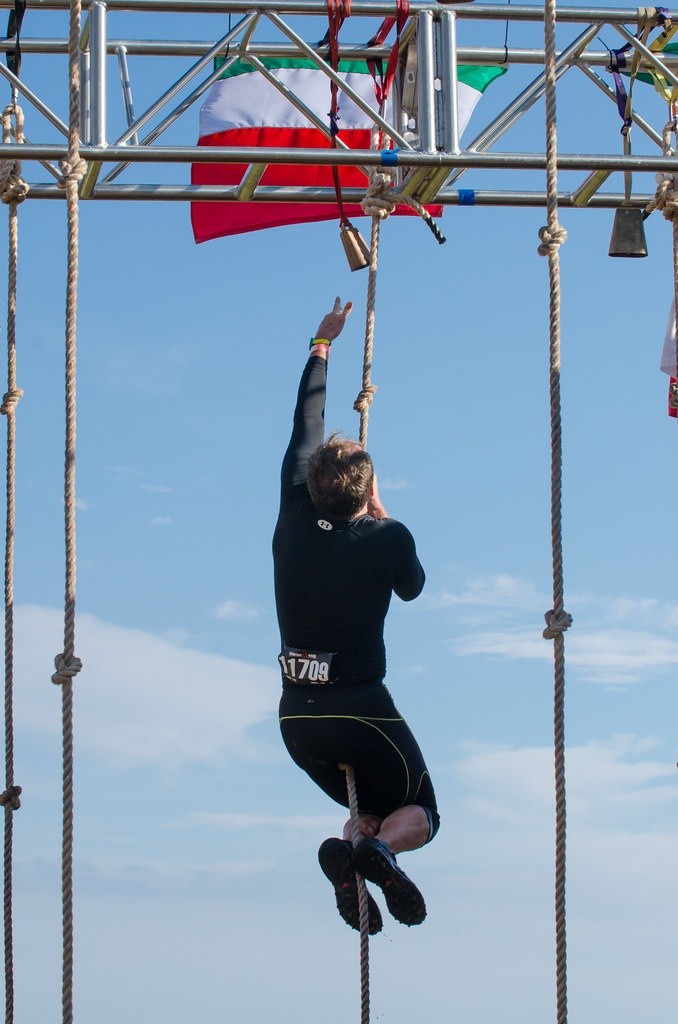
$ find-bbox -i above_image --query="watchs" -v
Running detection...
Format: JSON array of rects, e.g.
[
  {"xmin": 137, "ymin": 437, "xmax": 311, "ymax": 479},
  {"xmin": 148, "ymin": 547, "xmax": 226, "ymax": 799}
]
[{"xmin": 309, "ymin": 337, "xmax": 331, "ymax": 349}]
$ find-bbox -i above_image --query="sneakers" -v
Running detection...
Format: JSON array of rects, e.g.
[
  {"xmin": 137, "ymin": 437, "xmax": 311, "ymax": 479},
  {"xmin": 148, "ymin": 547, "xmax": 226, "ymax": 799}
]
[
  {"xmin": 349, "ymin": 835, "xmax": 427, "ymax": 928},
  {"xmin": 318, "ymin": 837, "xmax": 383, "ymax": 936}
]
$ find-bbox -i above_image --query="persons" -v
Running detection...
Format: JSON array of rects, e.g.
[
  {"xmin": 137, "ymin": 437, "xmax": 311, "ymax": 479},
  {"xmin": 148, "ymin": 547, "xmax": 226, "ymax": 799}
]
[{"xmin": 272, "ymin": 296, "xmax": 439, "ymax": 934}]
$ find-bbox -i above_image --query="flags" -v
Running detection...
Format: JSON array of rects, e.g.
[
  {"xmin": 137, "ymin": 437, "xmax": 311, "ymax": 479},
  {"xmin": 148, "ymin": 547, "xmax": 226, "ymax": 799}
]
[{"xmin": 187, "ymin": 54, "xmax": 508, "ymax": 245}]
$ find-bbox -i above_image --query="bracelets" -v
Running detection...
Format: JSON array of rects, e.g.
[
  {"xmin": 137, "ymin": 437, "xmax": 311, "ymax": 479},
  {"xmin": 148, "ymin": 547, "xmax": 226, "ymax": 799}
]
[{"xmin": 310, "ymin": 343, "xmax": 331, "ymax": 354}]
